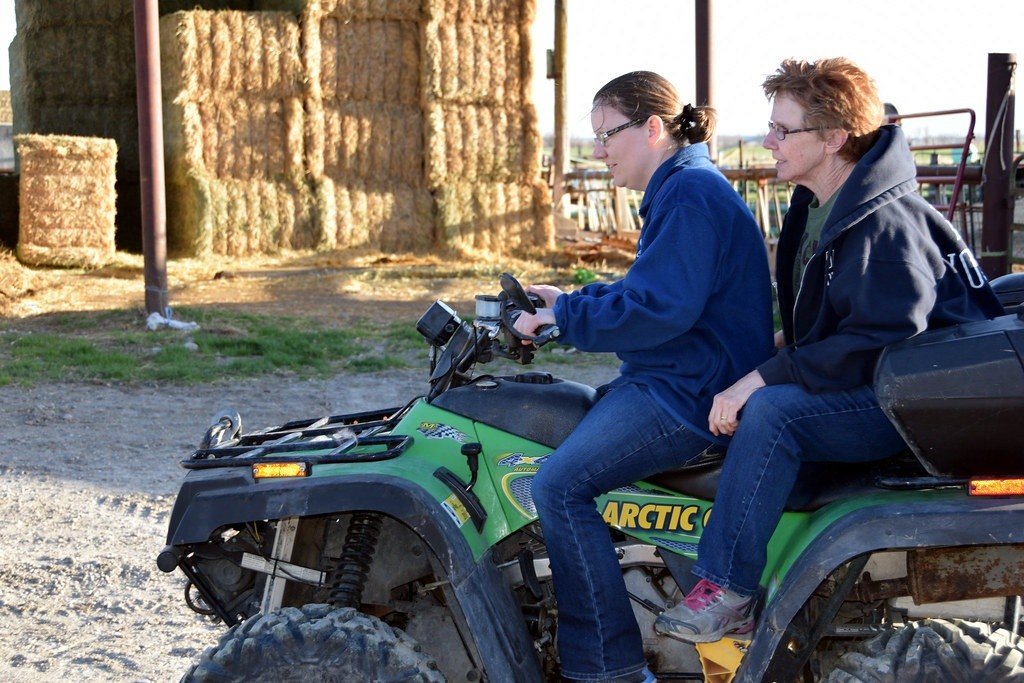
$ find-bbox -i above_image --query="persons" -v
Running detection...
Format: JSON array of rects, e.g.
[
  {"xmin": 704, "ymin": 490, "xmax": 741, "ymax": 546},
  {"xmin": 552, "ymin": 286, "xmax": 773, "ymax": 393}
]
[
  {"xmin": 654, "ymin": 56, "xmax": 1005, "ymax": 641},
  {"xmin": 514, "ymin": 72, "xmax": 773, "ymax": 683}
]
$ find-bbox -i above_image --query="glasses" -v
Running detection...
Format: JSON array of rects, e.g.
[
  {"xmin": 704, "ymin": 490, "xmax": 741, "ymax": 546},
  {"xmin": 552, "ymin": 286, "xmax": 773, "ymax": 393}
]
[
  {"xmin": 767, "ymin": 120, "xmax": 843, "ymax": 141},
  {"xmin": 594, "ymin": 116, "xmax": 648, "ymax": 146}
]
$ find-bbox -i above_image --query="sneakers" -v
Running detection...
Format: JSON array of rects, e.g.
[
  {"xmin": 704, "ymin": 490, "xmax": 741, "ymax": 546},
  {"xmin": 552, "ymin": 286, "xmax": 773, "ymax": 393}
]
[
  {"xmin": 579, "ymin": 664, "xmax": 659, "ymax": 683},
  {"xmin": 652, "ymin": 577, "xmax": 760, "ymax": 643}
]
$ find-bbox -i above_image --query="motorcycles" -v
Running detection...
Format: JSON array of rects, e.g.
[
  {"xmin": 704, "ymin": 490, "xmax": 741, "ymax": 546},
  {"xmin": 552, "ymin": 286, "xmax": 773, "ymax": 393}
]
[{"xmin": 156, "ymin": 273, "xmax": 1024, "ymax": 683}]
